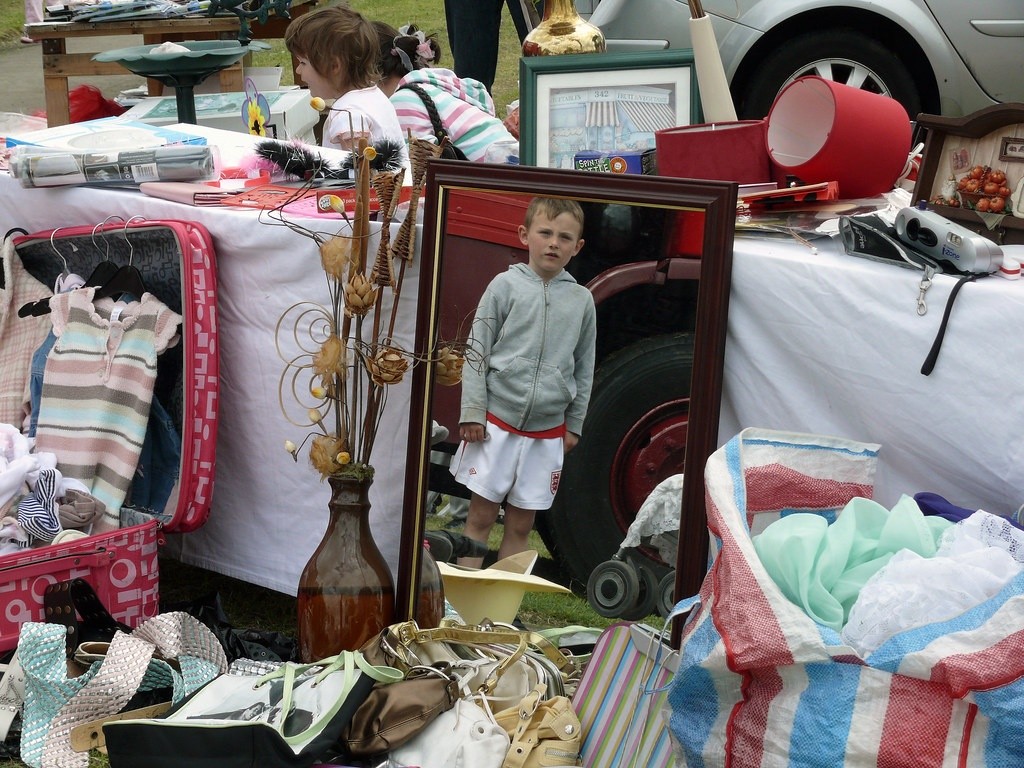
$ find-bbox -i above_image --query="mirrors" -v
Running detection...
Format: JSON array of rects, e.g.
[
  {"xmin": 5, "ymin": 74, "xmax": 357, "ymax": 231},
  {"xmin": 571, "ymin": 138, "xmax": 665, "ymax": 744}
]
[{"xmin": 391, "ymin": 156, "xmax": 740, "ymax": 658}]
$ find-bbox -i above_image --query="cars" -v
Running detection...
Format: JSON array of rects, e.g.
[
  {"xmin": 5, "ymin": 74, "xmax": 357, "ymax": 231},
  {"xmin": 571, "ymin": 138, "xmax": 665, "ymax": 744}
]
[{"xmin": 587, "ymin": 0, "xmax": 1024, "ymax": 155}]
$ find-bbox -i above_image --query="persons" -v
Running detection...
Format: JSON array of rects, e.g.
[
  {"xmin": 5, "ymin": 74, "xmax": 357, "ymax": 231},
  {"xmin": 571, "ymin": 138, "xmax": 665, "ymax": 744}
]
[
  {"xmin": 365, "ymin": 20, "xmax": 519, "ymax": 165},
  {"xmin": 284, "ymin": 5, "xmax": 414, "ymax": 187},
  {"xmin": 443, "ymin": 0, "xmax": 555, "ymax": 100},
  {"xmin": 451, "ymin": 195, "xmax": 598, "ymax": 560}
]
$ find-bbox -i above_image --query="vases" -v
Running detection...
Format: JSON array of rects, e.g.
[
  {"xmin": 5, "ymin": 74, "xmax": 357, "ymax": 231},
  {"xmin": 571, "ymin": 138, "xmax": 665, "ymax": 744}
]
[{"xmin": 295, "ymin": 469, "xmax": 395, "ymax": 665}]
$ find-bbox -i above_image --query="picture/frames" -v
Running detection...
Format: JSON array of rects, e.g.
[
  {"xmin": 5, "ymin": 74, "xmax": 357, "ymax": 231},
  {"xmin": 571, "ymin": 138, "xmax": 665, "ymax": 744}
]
[{"xmin": 519, "ymin": 48, "xmax": 703, "ymax": 175}]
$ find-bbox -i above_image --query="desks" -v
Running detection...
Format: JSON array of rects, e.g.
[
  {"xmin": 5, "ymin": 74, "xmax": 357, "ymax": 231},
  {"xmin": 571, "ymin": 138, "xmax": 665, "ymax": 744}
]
[
  {"xmin": 0, "ymin": 115, "xmax": 1023, "ymax": 596},
  {"xmin": 26, "ymin": 0, "xmax": 320, "ymax": 132}
]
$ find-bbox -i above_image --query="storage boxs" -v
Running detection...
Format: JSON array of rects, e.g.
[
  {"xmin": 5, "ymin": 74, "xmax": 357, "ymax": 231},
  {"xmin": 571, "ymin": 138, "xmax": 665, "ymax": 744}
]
[{"xmin": 124, "ymin": 85, "xmax": 319, "ymax": 146}]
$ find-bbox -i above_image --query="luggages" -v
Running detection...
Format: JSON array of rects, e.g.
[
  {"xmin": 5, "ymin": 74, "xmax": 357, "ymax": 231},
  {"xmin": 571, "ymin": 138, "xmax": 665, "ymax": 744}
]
[{"xmin": 0, "ymin": 220, "xmax": 218, "ymax": 651}]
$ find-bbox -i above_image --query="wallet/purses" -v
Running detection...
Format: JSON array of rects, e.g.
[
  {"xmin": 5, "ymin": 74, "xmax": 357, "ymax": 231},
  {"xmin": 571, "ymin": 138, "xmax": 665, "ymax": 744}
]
[{"xmin": 140, "ymin": 180, "xmax": 235, "ymax": 207}]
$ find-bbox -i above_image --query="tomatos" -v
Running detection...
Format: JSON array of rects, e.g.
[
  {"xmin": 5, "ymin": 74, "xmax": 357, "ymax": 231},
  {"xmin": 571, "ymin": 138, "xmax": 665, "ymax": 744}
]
[{"xmin": 948, "ymin": 165, "xmax": 1010, "ymax": 213}]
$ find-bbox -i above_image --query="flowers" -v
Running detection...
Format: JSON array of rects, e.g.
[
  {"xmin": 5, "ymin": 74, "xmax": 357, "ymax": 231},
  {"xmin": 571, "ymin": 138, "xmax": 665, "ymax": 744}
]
[{"xmin": 252, "ymin": 103, "xmax": 506, "ymax": 478}]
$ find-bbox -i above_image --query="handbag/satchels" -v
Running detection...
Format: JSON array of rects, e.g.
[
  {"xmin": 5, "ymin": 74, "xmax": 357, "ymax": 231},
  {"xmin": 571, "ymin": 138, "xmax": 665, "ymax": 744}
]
[
  {"xmin": 395, "ymin": 83, "xmax": 471, "ymax": 161},
  {"xmin": 103, "ymin": 578, "xmax": 583, "ymax": 768},
  {"xmin": 670, "ymin": 427, "xmax": 1022, "ymax": 766}
]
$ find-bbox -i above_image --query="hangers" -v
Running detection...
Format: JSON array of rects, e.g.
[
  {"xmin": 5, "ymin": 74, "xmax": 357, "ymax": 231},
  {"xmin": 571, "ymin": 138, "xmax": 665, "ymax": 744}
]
[{"xmin": 17, "ymin": 215, "xmax": 182, "ymax": 332}]
